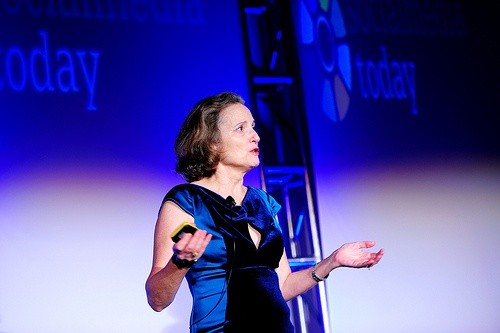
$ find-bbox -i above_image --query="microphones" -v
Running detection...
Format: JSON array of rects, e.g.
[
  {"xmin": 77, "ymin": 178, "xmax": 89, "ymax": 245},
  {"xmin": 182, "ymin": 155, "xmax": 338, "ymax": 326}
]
[{"xmin": 226, "ymin": 196, "xmax": 236, "ymax": 205}]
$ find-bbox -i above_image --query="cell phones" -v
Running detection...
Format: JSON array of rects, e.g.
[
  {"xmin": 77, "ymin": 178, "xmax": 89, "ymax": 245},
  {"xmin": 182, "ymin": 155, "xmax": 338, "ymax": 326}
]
[{"xmin": 170, "ymin": 222, "xmax": 199, "ymax": 244}]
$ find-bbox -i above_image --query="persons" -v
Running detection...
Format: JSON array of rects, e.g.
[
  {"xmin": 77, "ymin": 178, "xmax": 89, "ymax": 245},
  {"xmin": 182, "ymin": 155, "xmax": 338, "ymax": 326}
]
[{"xmin": 144, "ymin": 91, "xmax": 384, "ymax": 332}]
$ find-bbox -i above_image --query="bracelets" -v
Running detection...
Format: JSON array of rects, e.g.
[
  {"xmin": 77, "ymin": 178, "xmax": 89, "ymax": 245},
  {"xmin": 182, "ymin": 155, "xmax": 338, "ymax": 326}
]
[
  {"xmin": 172, "ymin": 255, "xmax": 195, "ymax": 267},
  {"xmin": 312, "ymin": 263, "xmax": 329, "ymax": 282}
]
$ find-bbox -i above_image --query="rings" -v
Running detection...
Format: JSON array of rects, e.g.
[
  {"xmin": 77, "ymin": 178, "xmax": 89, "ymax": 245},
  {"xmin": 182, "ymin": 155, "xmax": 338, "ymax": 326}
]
[{"xmin": 192, "ymin": 253, "xmax": 198, "ymax": 257}]
